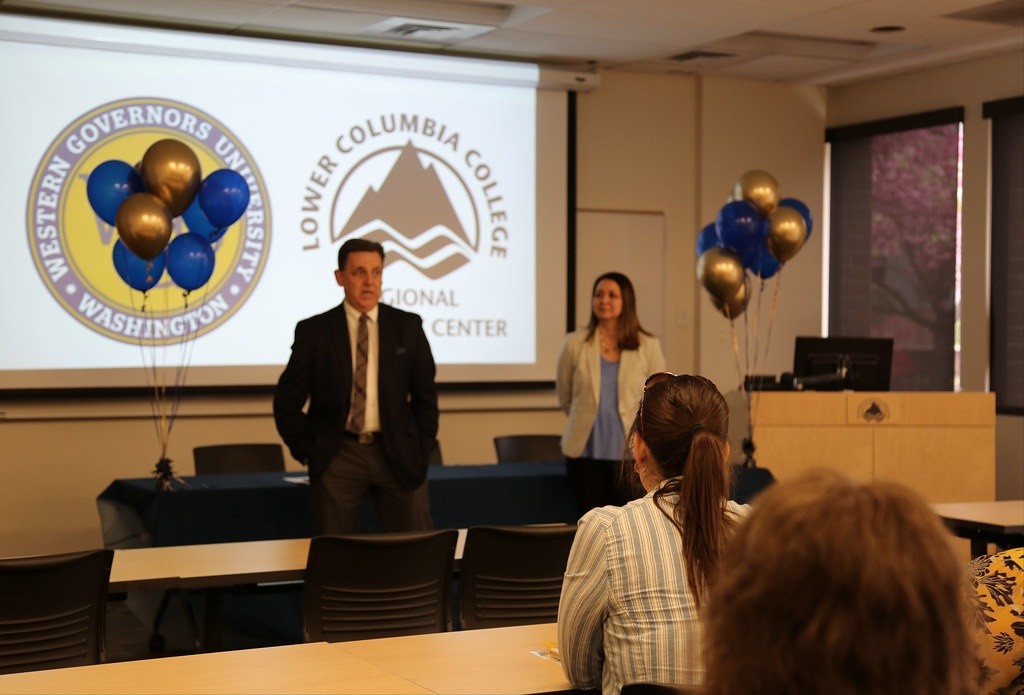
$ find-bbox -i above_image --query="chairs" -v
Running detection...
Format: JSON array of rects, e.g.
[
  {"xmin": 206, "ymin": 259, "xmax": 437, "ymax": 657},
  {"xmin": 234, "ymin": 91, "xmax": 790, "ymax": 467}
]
[
  {"xmin": 458, "ymin": 525, "xmax": 579, "ymax": 630},
  {"xmin": 496, "ymin": 434, "xmax": 565, "ymax": 462},
  {"xmin": 302, "ymin": 532, "xmax": 458, "ymax": 643},
  {"xmin": 0, "ymin": 546, "xmax": 113, "ymax": 675},
  {"xmin": 193, "ymin": 443, "xmax": 284, "ymax": 474}
]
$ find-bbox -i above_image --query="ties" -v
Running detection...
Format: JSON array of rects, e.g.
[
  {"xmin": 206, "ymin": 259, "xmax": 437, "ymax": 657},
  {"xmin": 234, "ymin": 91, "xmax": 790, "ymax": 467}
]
[{"xmin": 349, "ymin": 313, "xmax": 370, "ymax": 432}]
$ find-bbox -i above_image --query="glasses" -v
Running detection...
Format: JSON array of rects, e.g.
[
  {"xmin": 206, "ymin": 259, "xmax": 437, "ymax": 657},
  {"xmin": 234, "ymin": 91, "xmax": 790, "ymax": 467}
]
[{"xmin": 640, "ymin": 371, "xmax": 717, "ymax": 441}]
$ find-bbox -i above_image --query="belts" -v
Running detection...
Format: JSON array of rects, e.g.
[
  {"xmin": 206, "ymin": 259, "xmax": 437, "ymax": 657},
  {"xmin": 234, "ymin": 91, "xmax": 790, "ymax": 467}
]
[{"xmin": 342, "ymin": 430, "xmax": 383, "ymax": 445}]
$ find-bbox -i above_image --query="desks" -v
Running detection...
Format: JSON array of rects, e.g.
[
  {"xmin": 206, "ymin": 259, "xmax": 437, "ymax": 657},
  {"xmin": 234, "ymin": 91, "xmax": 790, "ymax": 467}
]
[
  {"xmin": 930, "ymin": 500, "xmax": 1024, "ymax": 561},
  {"xmin": 0, "ymin": 624, "xmax": 574, "ymax": 695},
  {"xmin": 108, "ymin": 528, "xmax": 464, "ymax": 592},
  {"xmin": 98, "ymin": 458, "xmax": 573, "ymax": 655}
]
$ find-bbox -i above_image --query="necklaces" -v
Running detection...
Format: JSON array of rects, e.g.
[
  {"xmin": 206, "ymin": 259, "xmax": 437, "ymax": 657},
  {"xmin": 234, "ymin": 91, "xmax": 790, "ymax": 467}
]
[{"xmin": 599, "ymin": 329, "xmax": 618, "ymax": 352}]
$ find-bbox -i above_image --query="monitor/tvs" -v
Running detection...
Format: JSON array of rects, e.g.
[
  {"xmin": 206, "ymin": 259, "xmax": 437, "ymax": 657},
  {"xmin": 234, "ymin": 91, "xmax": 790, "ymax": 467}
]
[{"xmin": 793, "ymin": 336, "xmax": 893, "ymax": 391}]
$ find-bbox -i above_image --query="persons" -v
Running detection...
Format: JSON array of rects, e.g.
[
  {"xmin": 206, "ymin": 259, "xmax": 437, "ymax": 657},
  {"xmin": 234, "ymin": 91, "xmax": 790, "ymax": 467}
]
[
  {"xmin": 780, "ymin": 371, "xmax": 793, "ymax": 389},
  {"xmin": 845, "ymin": 365, "xmax": 873, "ymax": 391},
  {"xmin": 555, "ymin": 272, "xmax": 667, "ymax": 511},
  {"xmin": 704, "ymin": 470, "xmax": 969, "ymax": 695},
  {"xmin": 557, "ymin": 374, "xmax": 752, "ymax": 695},
  {"xmin": 272, "ymin": 239, "xmax": 437, "ymax": 536}
]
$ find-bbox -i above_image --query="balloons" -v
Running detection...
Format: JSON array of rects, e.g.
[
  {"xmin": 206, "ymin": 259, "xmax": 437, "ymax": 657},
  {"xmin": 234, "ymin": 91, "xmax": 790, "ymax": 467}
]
[
  {"xmin": 88, "ymin": 137, "xmax": 250, "ymax": 292},
  {"xmin": 695, "ymin": 168, "xmax": 813, "ymax": 321}
]
